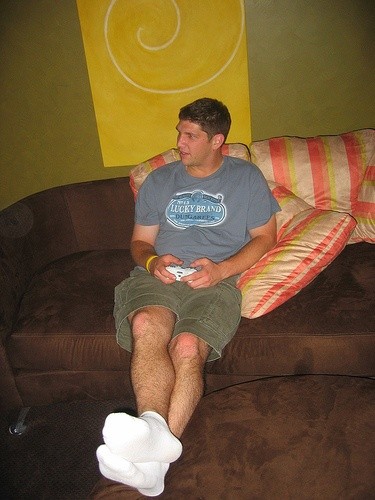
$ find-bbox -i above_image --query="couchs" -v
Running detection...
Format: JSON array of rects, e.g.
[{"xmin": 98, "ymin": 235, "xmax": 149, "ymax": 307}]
[{"xmin": 0, "ymin": 176, "xmax": 375, "ymax": 435}]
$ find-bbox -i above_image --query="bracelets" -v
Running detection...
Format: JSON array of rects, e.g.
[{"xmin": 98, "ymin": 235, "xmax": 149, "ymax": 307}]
[{"xmin": 146, "ymin": 255, "xmax": 158, "ymax": 274}]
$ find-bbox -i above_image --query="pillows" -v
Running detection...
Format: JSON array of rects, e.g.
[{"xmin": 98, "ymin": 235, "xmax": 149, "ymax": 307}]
[{"xmin": 130, "ymin": 129, "xmax": 375, "ymax": 318}]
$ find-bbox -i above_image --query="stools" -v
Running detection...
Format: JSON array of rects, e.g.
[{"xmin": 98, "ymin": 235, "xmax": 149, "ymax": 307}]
[{"xmin": 88, "ymin": 374, "xmax": 375, "ymax": 500}]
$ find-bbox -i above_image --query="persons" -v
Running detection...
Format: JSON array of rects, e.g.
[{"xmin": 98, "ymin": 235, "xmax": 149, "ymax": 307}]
[{"xmin": 95, "ymin": 97, "xmax": 282, "ymax": 497}]
